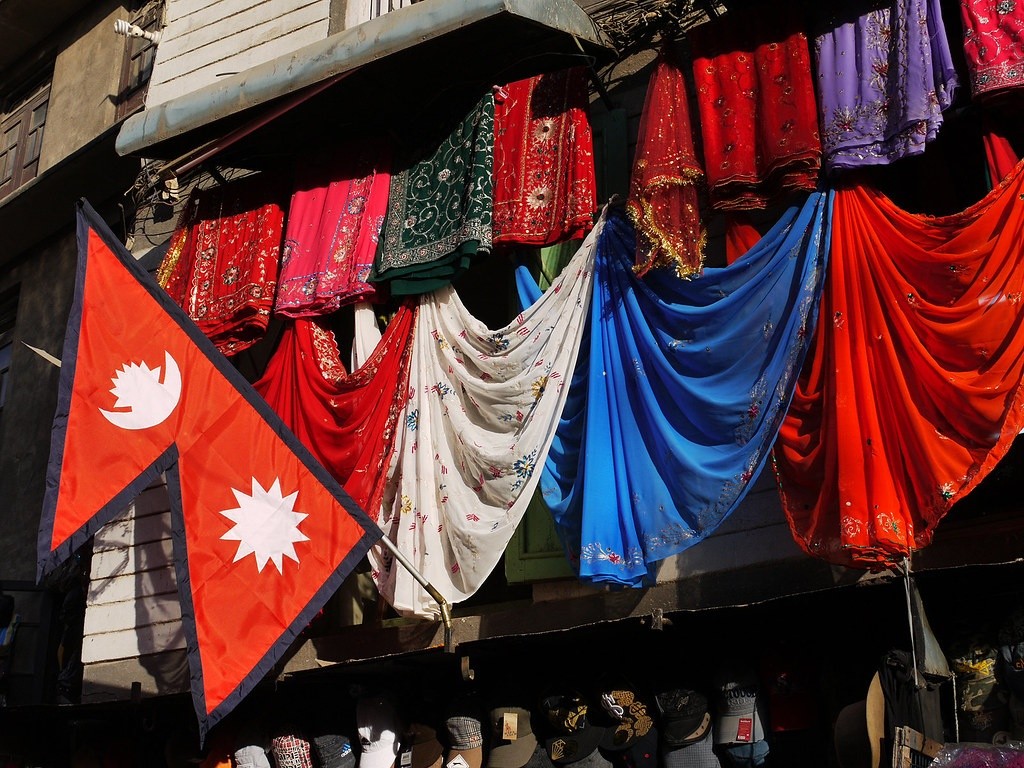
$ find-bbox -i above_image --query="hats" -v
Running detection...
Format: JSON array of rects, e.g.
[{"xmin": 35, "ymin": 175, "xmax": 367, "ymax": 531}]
[{"xmin": 201, "ymin": 636, "xmax": 834, "ymax": 768}]
